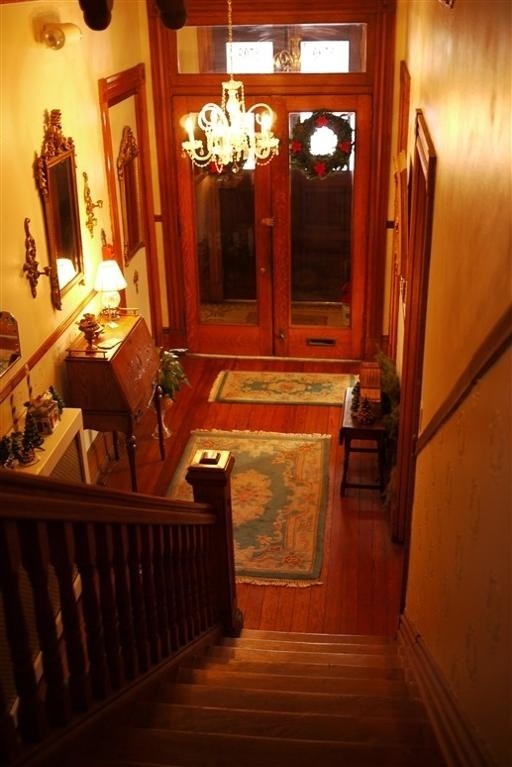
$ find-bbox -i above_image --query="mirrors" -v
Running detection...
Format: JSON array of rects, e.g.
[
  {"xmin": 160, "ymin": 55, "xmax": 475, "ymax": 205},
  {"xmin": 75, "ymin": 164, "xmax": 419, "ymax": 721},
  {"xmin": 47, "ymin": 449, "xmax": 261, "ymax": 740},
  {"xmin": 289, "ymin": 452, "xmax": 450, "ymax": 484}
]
[
  {"xmin": 24, "ymin": 110, "xmax": 86, "ymax": 310},
  {"xmin": 0, "ymin": 311, "xmax": 21, "ymax": 378}
]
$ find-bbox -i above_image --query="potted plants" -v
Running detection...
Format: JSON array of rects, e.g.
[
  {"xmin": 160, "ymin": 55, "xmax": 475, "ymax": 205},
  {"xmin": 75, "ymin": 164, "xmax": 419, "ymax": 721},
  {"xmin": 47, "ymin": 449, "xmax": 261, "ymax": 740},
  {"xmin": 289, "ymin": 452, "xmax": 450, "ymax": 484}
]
[{"xmin": 152, "ymin": 350, "xmax": 192, "ymax": 439}]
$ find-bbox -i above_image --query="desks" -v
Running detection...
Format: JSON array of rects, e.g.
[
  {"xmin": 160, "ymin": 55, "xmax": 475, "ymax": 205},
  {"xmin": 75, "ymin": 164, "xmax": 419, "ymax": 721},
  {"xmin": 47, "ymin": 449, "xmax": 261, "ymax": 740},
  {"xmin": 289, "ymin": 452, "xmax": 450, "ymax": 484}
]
[
  {"xmin": 63, "ymin": 307, "xmax": 165, "ymax": 492},
  {"xmin": 338, "ymin": 387, "xmax": 388, "ymax": 504}
]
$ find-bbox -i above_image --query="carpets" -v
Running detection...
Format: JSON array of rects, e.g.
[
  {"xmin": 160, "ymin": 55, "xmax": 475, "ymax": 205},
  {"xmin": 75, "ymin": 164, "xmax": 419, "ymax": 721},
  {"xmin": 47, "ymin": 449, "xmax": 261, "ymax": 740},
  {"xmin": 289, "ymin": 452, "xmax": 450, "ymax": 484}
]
[
  {"xmin": 201, "ymin": 303, "xmax": 347, "ymax": 326},
  {"xmin": 167, "ymin": 428, "xmax": 332, "ymax": 588},
  {"xmin": 208, "ymin": 370, "xmax": 359, "ymax": 406}
]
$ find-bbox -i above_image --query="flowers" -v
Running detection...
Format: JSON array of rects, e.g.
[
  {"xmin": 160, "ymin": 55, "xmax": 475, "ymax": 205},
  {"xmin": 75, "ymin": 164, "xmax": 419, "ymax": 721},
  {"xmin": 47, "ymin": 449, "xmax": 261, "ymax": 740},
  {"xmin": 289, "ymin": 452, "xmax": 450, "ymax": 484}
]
[{"xmin": 291, "ymin": 111, "xmax": 352, "ymax": 180}]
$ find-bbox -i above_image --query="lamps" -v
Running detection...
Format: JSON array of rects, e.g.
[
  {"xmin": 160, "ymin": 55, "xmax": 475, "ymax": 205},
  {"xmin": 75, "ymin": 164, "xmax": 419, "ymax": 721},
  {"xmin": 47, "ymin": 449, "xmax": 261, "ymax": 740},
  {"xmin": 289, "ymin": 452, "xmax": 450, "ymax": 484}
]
[
  {"xmin": 55, "ymin": 257, "xmax": 77, "ymax": 290},
  {"xmin": 276, "ymin": 25, "xmax": 302, "ymax": 72},
  {"xmin": 180, "ymin": 1, "xmax": 280, "ymax": 174},
  {"xmin": 94, "ymin": 259, "xmax": 127, "ymax": 328},
  {"xmin": 42, "ymin": 22, "xmax": 83, "ymax": 52}
]
